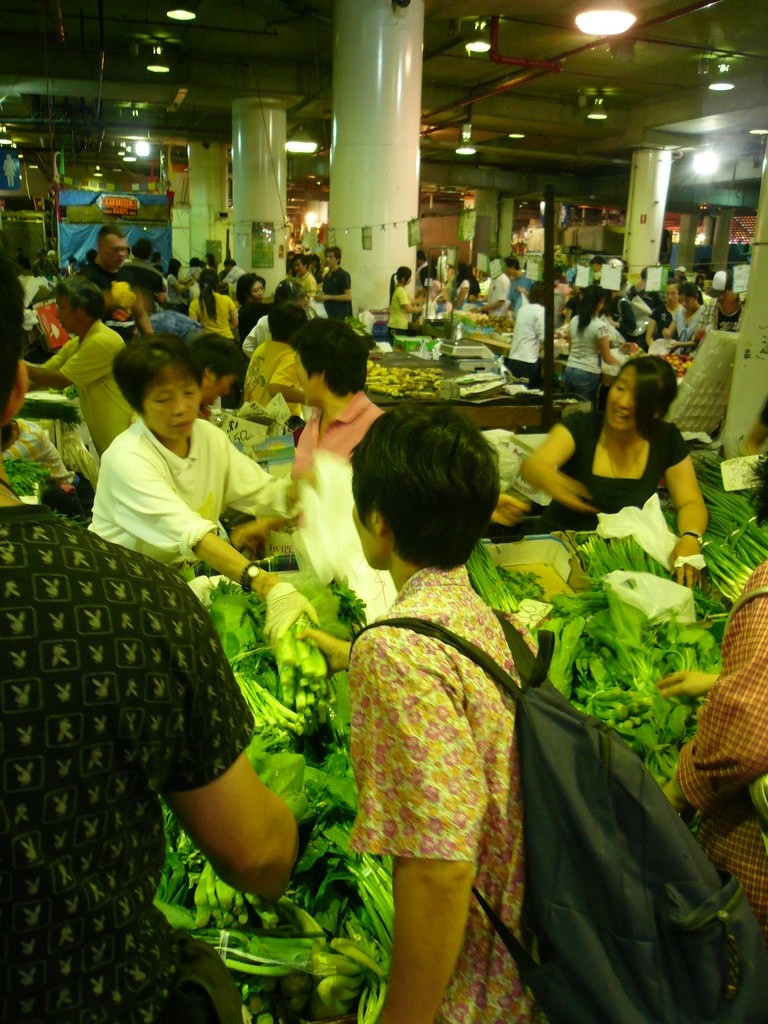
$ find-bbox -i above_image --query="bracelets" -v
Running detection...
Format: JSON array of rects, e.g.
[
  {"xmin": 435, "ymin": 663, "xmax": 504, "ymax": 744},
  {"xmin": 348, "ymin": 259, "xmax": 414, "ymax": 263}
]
[
  {"xmin": 240, "ymin": 561, "xmax": 258, "ymax": 593},
  {"xmin": 477, "ymin": 307, "xmax": 481, "ymax": 311},
  {"xmin": 683, "ymin": 530, "xmax": 704, "ymax": 545}
]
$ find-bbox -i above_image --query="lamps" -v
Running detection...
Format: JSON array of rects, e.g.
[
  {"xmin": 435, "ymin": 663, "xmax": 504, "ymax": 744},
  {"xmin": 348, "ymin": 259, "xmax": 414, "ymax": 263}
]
[
  {"xmin": 456, "ymin": 123, "xmax": 476, "ymax": 155},
  {"xmin": 165, "ymin": 2, "xmax": 199, "ymax": 20},
  {"xmin": 146, "ymin": 38, "xmax": 170, "ymax": 74}
]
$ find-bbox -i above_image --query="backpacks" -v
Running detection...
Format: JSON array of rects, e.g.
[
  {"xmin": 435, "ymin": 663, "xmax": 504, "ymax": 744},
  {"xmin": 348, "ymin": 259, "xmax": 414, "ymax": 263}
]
[{"xmin": 349, "ymin": 606, "xmax": 768, "ymax": 1023}]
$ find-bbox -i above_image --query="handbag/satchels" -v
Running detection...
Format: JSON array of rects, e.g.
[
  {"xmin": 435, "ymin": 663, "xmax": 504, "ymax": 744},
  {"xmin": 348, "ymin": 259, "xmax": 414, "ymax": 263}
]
[{"xmin": 616, "ymin": 294, "xmax": 652, "ymax": 336}]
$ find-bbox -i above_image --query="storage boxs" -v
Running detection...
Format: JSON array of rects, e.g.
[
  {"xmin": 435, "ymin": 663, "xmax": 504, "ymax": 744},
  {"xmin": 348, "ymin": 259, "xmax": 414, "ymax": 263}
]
[
  {"xmin": 479, "ymin": 529, "xmax": 587, "ymax": 605},
  {"xmin": 392, "ymin": 334, "xmax": 437, "ymax": 351}
]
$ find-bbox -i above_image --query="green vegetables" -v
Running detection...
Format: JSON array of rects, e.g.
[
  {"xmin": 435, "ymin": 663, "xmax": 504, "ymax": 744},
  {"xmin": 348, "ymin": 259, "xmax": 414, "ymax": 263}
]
[
  {"xmin": 2, "ymin": 456, "xmax": 52, "ymax": 496},
  {"xmin": 13, "ymin": 385, "xmax": 84, "ymax": 432},
  {"xmin": 537, "ymin": 586, "xmax": 725, "ymax": 837},
  {"xmin": 579, "ymin": 535, "xmax": 676, "ymax": 581},
  {"xmin": 154, "ymin": 572, "xmax": 402, "ymax": 1024},
  {"xmin": 465, "ymin": 538, "xmax": 544, "ymax": 615}
]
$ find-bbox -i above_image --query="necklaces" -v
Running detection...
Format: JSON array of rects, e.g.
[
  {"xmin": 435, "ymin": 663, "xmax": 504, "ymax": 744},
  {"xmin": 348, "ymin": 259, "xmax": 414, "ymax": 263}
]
[{"xmin": 605, "ymin": 427, "xmax": 646, "ymax": 479}]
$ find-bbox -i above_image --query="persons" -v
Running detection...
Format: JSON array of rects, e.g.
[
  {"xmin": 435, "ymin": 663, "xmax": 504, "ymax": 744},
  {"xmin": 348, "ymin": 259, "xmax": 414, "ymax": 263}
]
[
  {"xmin": 387, "ymin": 267, "xmax": 421, "ymax": 346},
  {"xmin": 84, "ymin": 332, "xmax": 321, "ymax": 640},
  {"xmin": 742, "ymin": 395, "xmax": 768, "ymax": 455},
  {"xmin": 447, "ymin": 253, "xmax": 532, "ymax": 318},
  {"xmin": 21, "ymin": 272, "xmax": 138, "ymax": 459},
  {"xmin": 522, "ymin": 351, "xmax": 708, "ymax": 589},
  {"xmin": 414, "ymin": 251, "xmax": 437, "ymax": 297},
  {"xmin": 506, "ymin": 281, "xmax": 546, "ymax": 379},
  {"xmin": 135, "ymin": 251, "xmax": 246, "ymax": 409},
  {"xmin": 1, "ymin": 417, "xmax": 75, "ymax": 484},
  {"xmin": 234, "ymin": 246, "xmax": 354, "ymax": 355},
  {"xmin": 300, "ymin": 406, "xmax": 549, "ymax": 1024},
  {"xmin": 60, "ymin": 223, "xmax": 168, "ymax": 344},
  {"xmin": 1, "ymin": 259, "xmax": 300, "ymax": 1024},
  {"xmin": 562, "ymin": 284, "xmax": 625, "ymax": 399},
  {"xmin": 653, "ymin": 448, "xmax": 766, "ymax": 932},
  {"xmin": 230, "ymin": 315, "xmax": 399, "ymax": 625},
  {"xmin": 563, "ymin": 254, "xmax": 747, "ymax": 354},
  {"xmin": 241, "ymin": 303, "xmax": 309, "ymax": 417}
]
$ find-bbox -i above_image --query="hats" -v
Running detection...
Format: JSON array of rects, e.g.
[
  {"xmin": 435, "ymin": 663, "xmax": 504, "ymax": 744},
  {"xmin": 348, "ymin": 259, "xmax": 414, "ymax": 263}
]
[
  {"xmin": 705, "ymin": 270, "xmax": 734, "ymax": 298},
  {"xmin": 675, "ymin": 267, "xmax": 685, "ymax": 272}
]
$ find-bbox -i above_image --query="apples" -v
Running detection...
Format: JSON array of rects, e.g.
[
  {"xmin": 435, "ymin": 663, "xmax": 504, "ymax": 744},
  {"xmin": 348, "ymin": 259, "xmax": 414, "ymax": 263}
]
[{"xmin": 662, "ymin": 353, "xmax": 693, "ymax": 376}]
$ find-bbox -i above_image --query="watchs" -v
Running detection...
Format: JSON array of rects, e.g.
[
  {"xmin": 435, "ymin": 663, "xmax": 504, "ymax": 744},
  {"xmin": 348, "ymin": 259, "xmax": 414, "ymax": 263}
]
[{"xmin": 245, "ymin": 564, "xmax": 261, "ymax": 591}]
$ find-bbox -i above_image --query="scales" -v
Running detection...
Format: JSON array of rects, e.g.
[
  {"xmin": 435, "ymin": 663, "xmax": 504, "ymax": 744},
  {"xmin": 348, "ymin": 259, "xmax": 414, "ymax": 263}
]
[{"xmin": 439, "ymin": 340, "xmax": 495, "ymax": 359}]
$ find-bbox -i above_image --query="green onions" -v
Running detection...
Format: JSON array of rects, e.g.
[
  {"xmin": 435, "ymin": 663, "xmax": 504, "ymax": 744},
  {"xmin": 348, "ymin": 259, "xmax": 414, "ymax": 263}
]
[{"xmin": 659, "ymin": 450, "xmax": 768, "ymax": 605}]
